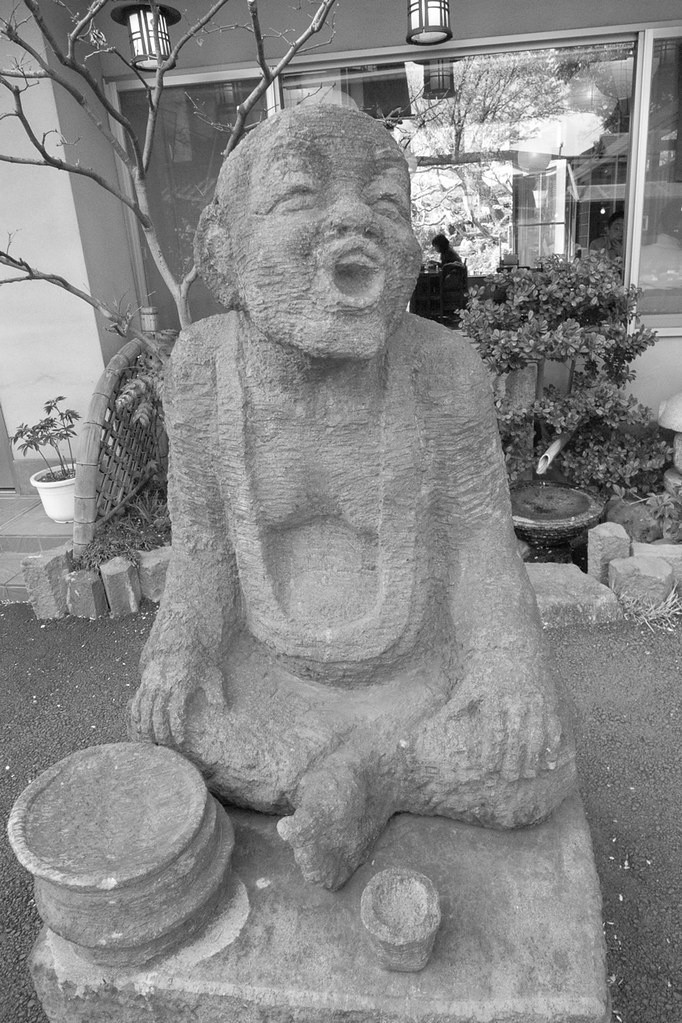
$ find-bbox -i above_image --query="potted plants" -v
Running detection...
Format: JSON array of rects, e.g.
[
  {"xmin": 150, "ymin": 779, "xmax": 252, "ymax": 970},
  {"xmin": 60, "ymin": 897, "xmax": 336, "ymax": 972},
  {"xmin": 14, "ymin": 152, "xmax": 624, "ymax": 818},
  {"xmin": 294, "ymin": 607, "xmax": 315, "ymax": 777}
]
[{"xmin": 7, "ymin": 395, "xmax": 82, "ymax": 525}]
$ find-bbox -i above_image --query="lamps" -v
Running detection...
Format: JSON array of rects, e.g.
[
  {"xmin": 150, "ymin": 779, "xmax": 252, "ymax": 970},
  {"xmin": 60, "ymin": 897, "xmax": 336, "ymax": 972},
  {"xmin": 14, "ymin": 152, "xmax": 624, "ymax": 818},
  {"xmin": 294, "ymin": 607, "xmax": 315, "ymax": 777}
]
[
  {"xmin": 417, "ymin": 57, "xmax": 462, "ymax": 100},
  {"xmin": 406, "ymin": 0, "xmax": 453, "ymax": 46},
  {"xmin": 113, "ymin": 0, "xmax": 178, "ymax": 71}
]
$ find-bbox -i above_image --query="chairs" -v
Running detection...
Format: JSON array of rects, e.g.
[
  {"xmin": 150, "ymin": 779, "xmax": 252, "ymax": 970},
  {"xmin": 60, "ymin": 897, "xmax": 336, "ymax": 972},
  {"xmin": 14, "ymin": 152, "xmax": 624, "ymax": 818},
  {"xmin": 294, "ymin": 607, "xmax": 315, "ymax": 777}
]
[{"xmin": 409, "ymin": 265, "xmax": 468, "ymax": 326}]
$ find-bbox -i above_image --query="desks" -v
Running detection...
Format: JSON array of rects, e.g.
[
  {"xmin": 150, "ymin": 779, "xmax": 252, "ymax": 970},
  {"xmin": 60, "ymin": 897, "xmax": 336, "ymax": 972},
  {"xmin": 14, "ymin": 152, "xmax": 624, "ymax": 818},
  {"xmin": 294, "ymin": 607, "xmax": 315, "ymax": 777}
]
[{"xmin": 414, "ymin": 268, "xmax": 445, "ymax": 321}]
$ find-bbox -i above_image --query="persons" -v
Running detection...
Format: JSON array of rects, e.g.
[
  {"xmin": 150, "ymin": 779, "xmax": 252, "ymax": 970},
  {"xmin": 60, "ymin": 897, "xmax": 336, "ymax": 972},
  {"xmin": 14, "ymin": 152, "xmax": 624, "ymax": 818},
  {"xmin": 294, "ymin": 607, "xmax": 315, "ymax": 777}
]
[
  {"xmin": 431, "ymin": 234, "xmax": 463, "ymax": 291},
  {"xmin": 634, "ymin": 196, "xmax": 682, "ymax": 289},
  {"xmin": 587, "ymin": 212, "xmax": 624, "ymax": 278},
  {"xmin": 128, "ymin": 101, "xmax": 576, "ymax": 895}
]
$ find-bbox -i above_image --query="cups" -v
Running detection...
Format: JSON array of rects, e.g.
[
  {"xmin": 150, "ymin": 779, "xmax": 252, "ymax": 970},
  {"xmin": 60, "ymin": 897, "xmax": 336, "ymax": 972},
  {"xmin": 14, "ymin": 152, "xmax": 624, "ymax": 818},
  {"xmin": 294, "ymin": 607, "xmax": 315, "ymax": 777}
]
[{"xmin": 359, "ymin": 865, "xmax": 441, "ymax": 973}]
